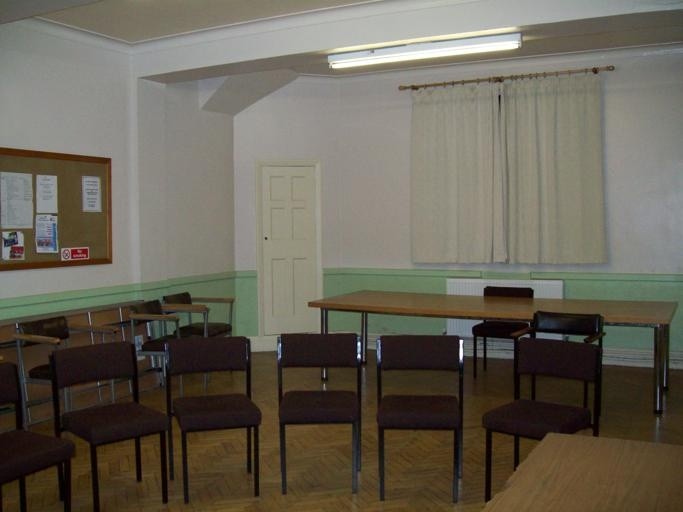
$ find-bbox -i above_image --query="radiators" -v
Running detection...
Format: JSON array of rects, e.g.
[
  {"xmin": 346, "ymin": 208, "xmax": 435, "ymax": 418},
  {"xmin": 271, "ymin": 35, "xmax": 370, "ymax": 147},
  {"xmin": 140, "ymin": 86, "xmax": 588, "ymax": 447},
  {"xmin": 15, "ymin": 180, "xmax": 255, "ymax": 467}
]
[{"xmin": 445, "ymin": 277, "xmax": 564, "ymax": 351}]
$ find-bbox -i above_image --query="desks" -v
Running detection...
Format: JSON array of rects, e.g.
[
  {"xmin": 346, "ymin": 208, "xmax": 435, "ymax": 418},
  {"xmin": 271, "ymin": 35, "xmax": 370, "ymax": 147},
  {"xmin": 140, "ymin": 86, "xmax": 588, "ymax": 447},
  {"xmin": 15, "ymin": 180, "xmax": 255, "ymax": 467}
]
[
  {"xmin": 477, "ymin": 431, "xmax": 682, "ymax": 512},
  {"xmin": 306, "ymin": 289, "xmax": 679, "ymax": 416}
]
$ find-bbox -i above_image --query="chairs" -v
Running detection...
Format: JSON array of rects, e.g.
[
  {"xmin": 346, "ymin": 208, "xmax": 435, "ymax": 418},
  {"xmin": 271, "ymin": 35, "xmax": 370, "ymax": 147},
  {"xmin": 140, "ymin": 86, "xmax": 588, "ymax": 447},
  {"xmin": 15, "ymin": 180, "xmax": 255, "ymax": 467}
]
[
  {"xmin": 482, "ymin": 335, "xmax": 603, "ymax": 503},
  {"xmin": 276, "ymin": 333, "xmax": 363, "ymax": 494},
  {"xmin": 47, "ymin": 341, "xmax": 168, "ymax": 511},
  {"xmin": 163, "ymin": 335, "xmax": 263, "ymax": 502},
  {"xmin": 0, "ymin": 361, "xmax": 74, "ymax": 511},
  {"xmin": 375, "ymin": 334, "xmax": 464, "ymax": 502},
  {"xmin": 161, "ymin": 291, "xmax": 235, "ymax": 391},
  {"xmin": 510, "ymin": 310, "xmax": 606, "ymax": 407},
  {"xmin": 8, "ymin": 315, "xmax": 121, "ymax": 432},
  {"xmin": 127, "ymin": 299, "xmax": 209, "ymax": 398},
  {"xmin": 472, "ymin": 285, "xmax": 534, "ymax": 377}
]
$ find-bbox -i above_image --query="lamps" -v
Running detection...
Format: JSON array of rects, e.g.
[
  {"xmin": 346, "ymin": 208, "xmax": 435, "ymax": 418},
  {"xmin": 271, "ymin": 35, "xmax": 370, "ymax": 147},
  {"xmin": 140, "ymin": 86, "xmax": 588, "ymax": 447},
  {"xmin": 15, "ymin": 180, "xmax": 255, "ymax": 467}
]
[{"xmin": 327, "ymin": 32, "xmax": 523, "ymax": 70}]
